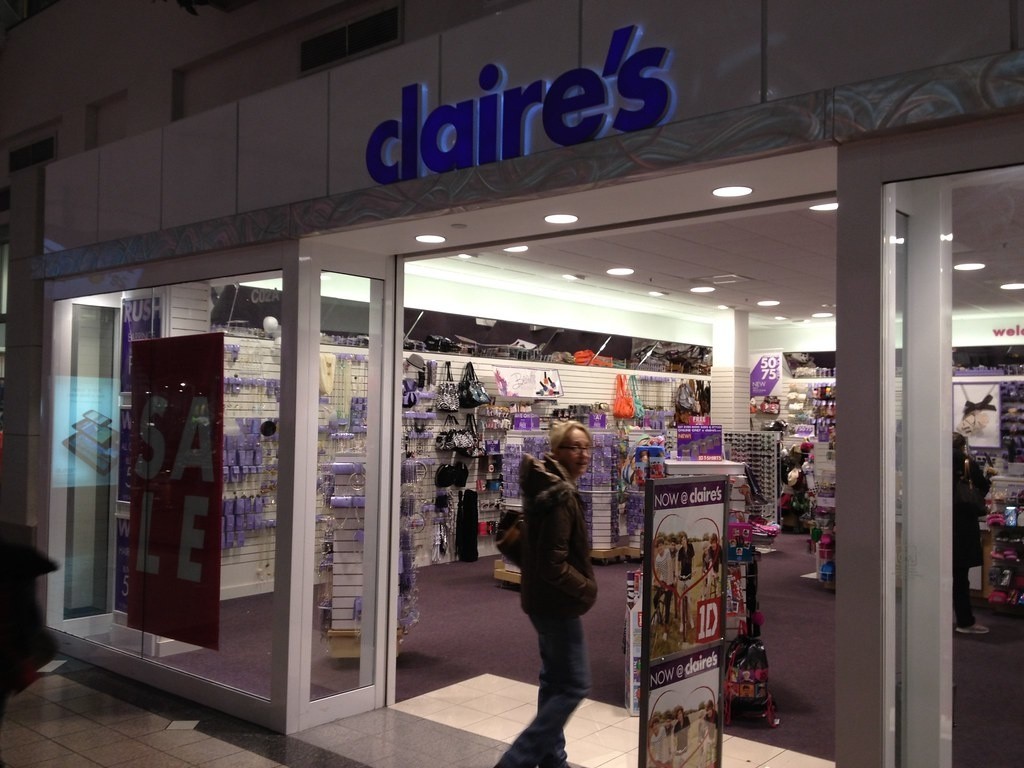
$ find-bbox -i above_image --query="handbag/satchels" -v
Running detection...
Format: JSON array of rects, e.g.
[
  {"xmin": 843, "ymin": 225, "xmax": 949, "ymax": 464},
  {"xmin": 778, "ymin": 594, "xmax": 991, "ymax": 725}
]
[
  {"xmin": 494, "ymin": 510, "xmax": 526, "ymax": 570},
  {"xmin": 467, "ymin": 413, "xmax": 485, "ymax": 457},
  {"xmin": 614, "ymin": 375, "xmax": 645, "ymax": 418},
  {"xmin": 955, "ymin": 458, "xmax": 978, "ymax": 502},
  {"xmin": 458, "ymin": 361, "xmax": 491, "ymax": 407},
  {"xmin": 436, "ymin": 415, "xmax": 474, "ymax": 451},
  {"xmin": 436, "ymin": 362, "xmax": 459, "ymax": 411}
]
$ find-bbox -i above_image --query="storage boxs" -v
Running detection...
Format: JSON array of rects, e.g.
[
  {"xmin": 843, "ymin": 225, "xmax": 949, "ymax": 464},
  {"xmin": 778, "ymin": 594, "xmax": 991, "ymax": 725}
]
[{"xmin": 727, "ymin": 523, "xmax": 753, "ymax": 543}]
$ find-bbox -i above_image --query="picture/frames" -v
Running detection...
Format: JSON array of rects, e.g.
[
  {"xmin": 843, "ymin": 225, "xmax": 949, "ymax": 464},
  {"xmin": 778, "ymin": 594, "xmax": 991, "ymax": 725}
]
[{"xmin": 952, "ymin": 382, "xmax": 1001, "ymax": 448}]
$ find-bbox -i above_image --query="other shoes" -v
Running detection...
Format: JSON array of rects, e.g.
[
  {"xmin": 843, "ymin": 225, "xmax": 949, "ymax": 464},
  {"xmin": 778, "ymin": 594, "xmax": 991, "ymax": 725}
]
[{"xmin": 956, "ymin": 620, "xmax": 989, "ymax": 634}]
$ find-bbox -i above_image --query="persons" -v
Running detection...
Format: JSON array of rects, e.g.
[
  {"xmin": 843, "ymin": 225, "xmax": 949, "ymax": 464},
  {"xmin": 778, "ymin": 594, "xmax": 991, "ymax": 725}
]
[
  {"xmin": 653, "ymin": 534, "xmax": 724, "ymax": 640},
  {"xmin": 494, "ymin": 421, "xmax": 600, "ymax": 768},
  {"xmin": 952, "ymin": 429, "xmax": 991, "ymax": 635},
  {"xmin": 649, "ymin": 698, "xmax": 719, "ymax": 768}
]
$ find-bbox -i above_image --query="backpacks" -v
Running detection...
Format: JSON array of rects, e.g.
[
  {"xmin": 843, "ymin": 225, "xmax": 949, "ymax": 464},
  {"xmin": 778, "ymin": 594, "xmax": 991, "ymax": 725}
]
[
  {"xmin": 675, "ymin": 383, "xmax": 695, "ymax": 412},
  {"xmin": 435, "ymin": 464, "xmax": 455, "ymax": 488},
  {"xmin": 453, "ymin": 461, "xmax": 468, "ymax": 487}
]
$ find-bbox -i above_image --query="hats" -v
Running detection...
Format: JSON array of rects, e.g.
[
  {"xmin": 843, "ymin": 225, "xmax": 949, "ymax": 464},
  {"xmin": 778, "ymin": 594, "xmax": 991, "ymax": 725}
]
[{"xmin": 787, "ymin": 468, "xmax": 799, "ymax": 486}]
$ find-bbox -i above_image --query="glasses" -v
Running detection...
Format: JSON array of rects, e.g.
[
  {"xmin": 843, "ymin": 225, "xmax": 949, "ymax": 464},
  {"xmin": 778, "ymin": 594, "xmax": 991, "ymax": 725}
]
[
  {"xmin": 559, "ymin": 445, "xmax": 594, "ymax": 452},
  {"xmin": 725, "ymin": 432, "xmax": 775, "ymax": 520}
]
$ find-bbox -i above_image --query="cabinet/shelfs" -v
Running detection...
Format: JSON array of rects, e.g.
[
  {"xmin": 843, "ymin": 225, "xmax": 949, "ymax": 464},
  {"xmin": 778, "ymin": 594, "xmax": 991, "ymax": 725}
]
[{"xmin": 989, "ymin": 477, "xmax": 1024, "ymax": 617}]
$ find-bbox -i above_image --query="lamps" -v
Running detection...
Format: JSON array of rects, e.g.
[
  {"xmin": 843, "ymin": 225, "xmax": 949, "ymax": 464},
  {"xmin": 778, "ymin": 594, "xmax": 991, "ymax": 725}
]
[{"xmin": 251, "ymin": 296, "xmax": 278, "ymax": 333}]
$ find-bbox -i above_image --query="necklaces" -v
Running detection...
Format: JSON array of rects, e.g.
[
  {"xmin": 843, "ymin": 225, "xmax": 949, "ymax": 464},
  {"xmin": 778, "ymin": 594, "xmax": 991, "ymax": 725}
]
[
  {"xmin": 433, "ymin": 491, "xmax": 455, "ymax": 563},
  {"xmin": 398, "ymin": 409, "xmax": 433, "ymax": 638}
]
